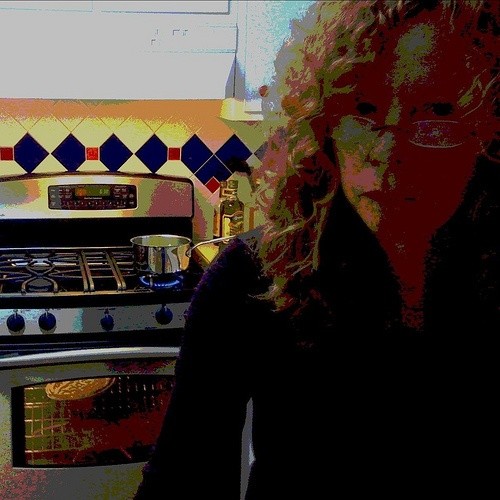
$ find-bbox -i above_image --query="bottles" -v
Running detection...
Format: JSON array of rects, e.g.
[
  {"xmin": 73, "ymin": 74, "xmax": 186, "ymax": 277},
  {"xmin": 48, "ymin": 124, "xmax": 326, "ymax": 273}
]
[
  {"xmin": 220, "ymin": 180, "xmax": 244, "ymax": 243},
  {"xmin": 212, "ymin": 181, "xmax": 226, "ymax": 244}
]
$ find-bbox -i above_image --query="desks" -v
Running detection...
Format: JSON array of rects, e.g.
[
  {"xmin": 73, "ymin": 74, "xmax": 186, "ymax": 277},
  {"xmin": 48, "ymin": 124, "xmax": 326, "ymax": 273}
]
[{"xmin": 191, "ymin": 239, "xmax": 219, "ymax": 272}]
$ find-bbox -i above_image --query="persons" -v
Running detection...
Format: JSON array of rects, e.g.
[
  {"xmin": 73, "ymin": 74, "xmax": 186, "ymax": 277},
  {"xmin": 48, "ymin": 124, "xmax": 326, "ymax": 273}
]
[{"xmin": 133, "ymin": 0, "xmax": 500, "ymax": 499}]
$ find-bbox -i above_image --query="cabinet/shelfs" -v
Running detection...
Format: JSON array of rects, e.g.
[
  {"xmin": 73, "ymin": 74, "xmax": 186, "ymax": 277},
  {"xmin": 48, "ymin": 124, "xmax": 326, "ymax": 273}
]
[{"xmin": 220, "ymin": 0, "xmax": 312, "ymax": 123}]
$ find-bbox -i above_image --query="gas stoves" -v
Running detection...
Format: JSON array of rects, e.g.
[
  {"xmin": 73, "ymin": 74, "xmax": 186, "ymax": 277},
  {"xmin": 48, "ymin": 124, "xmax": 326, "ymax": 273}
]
[{"xmin": 0, "ymin": 242, "xmax": 205, "ymax": 299}]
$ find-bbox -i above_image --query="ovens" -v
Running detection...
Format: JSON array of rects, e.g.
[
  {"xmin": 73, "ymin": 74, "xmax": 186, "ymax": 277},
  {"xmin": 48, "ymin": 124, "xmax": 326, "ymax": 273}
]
[{"xmin": 0, "ymin": 346, "xmax": 254, "ymax": 500}]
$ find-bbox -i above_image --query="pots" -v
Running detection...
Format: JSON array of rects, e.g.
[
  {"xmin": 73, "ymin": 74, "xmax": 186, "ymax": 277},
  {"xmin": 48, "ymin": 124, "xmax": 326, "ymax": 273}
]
[{"xmin": 131, "ymin": 234, "xmax": 233, "ymax": 273}]
